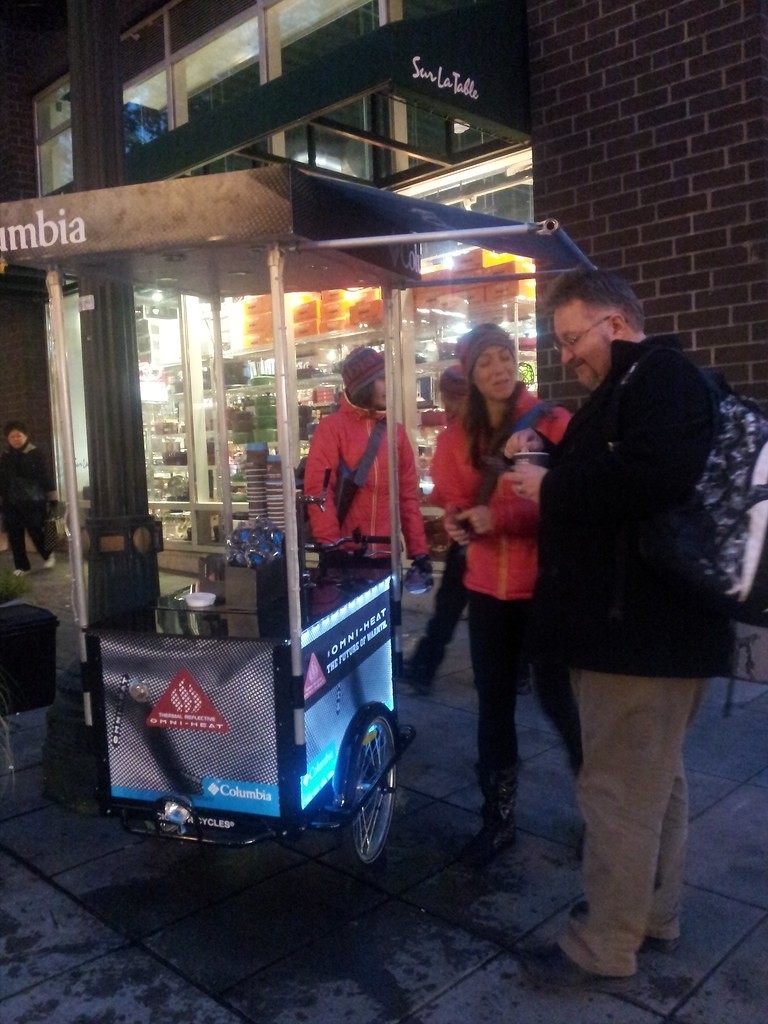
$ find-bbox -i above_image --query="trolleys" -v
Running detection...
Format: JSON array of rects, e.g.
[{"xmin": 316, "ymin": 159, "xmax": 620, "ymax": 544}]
[{"xmin": 79, "ymin": 523, "xmax": 436, "ymax": 869}]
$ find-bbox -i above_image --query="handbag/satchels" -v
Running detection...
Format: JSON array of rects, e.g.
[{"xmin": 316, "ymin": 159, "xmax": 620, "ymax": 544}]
[{"xmin": 42, "ymin": 517, "xmax": 67, "ymax": 548}]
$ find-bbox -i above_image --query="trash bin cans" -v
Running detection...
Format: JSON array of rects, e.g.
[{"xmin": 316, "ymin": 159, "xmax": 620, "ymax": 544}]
[{"xmin": 0, "ymin": 603, "xmax": 61, "ymax": 717}]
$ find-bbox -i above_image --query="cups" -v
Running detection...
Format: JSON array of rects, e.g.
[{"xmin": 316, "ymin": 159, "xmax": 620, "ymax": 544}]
[
  {"xmin": 185, "ymin": 593, "xmax": 217, "ymax": 611},
  {"xmin": 513, "ymin": 452, "xmax": 551, "ymax": 468},
  {"xmin": 245, "ymin": 442, "xmax": 286, "ymax": 534}
]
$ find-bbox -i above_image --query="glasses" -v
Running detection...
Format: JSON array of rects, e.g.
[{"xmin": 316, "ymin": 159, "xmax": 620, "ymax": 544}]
[{"xmin": 552, "ymin": 312, "xmax": 612, "ymax": 350}]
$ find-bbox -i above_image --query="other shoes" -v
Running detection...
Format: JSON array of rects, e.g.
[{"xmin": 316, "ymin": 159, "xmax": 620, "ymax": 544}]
[
  {"xmin": 44, "ymin": 551, "xmax": 54, "ymax": 566},
  {"xmin": 14, "ymin": 569, "xmax": 33, "ymax": 575},
  {"xmin": 520, "ymin": 943, "xmax": 602, "ymax": 986}
]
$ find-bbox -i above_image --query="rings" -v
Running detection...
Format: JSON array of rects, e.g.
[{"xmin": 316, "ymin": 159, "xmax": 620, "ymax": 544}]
[{"xmin": 518, "ymin": 484, "xmax": 523, "ymax": 494}]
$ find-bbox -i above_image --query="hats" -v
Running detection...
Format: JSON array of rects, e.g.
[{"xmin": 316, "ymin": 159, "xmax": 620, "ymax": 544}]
[
  {"xmin": 341, "ymin": 347, "xmax": 385, "ymax": 398},
  {"xmin": 440, "ymin": 366, "xmax": 472, "ymax": 399},
  {"xmin": 456, "ymin": 323, "xmax": 516, "ymax": 382}
]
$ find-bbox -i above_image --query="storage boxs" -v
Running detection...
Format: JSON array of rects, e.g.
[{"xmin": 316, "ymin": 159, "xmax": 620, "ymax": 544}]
[
  {"xmin": 239, "ymin": 250, "xmax": 535, "ymax": 348},
  {"xmin": 0, "ymin": 598, "xmax": 62, "ymax": 716}
]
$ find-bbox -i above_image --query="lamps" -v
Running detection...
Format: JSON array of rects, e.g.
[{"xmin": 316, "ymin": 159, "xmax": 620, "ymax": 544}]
[{"xmin": 130, "ymin": 33, "xmax": 140, "ymax": 41}]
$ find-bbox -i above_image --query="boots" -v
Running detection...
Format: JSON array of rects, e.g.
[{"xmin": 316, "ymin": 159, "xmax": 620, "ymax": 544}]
[
  {"xmin": 393, "ymin": 636, "xmax": 446, "ymax": 695},
  {"xmin": 456, "ymin": 786, "xmax": 517, "ymax": 870}
]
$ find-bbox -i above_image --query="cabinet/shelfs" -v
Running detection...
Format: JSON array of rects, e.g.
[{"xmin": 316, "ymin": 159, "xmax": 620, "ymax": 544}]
[{"xmin": 150, "ymin": 297, "xmax": 536, "ymax": 521}]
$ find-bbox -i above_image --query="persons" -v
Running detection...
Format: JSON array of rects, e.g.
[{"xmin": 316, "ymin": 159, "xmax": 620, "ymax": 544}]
[
  {"xmin": 399, "ymin": 363, "xmax": 532, "ymax": 695},
  {"xmin": 433, "ymin": 323, "xmax": 583, "ymax": 864},
  {"xmin": 228, "ymin": 450, "xmax": 240, "ymax": 476},
  {"xmin": 503, "ymin": 265, "xmax": 737, "ymax": 989},
  {"xmin": 0, "ymin": 420, "xmax": 58, "ymax": 577},
  {"xmin": 304, "ymin": 347, "xmax": 434, "ymax": 602}
]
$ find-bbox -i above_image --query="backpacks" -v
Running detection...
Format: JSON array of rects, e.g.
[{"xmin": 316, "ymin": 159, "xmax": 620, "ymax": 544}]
[{"xmin": 654, "ymin": 393, "xmax": 768, "ymax": 626}]
